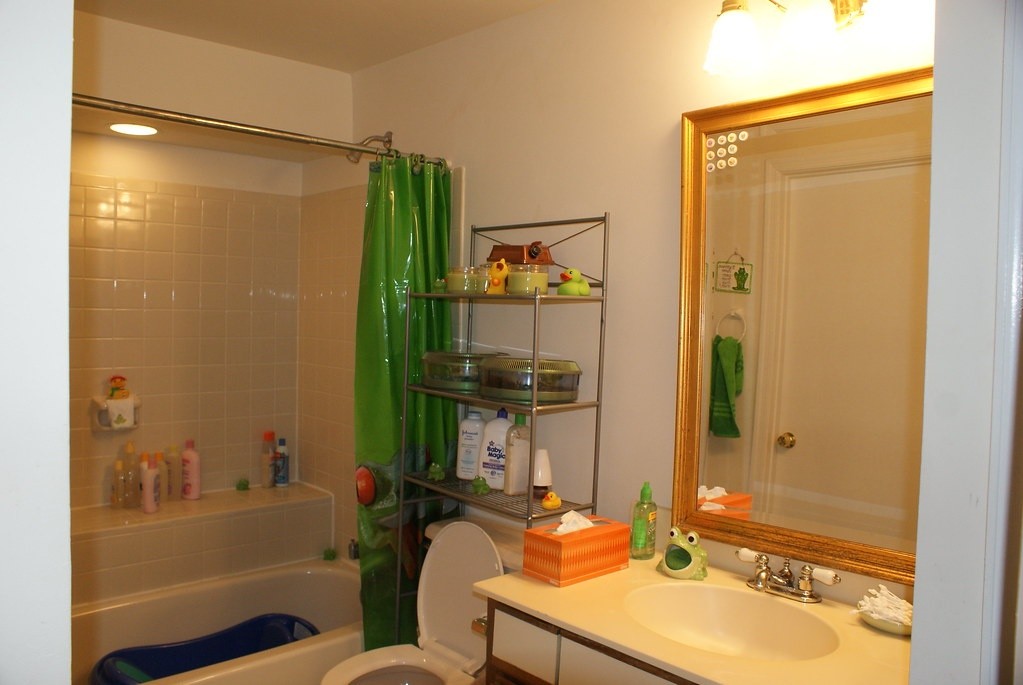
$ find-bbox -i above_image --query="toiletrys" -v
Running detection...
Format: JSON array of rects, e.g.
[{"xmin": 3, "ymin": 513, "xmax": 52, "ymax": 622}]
[
  {"xmin": 455, "ymin": 406, "xmax": 531, "ymax": 496},
  {"xmin": 109, "ymin": 429, "xmax": 290, "ymax": 515},
  {"xmin": 631, "ymin": 480, "xmax": 657, "ymax": 561}
]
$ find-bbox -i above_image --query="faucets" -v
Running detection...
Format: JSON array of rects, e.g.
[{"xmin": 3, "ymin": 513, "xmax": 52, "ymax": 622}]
[{"xmin": 767, "ymin": 556, "xmax": 795, "ymax": 597}]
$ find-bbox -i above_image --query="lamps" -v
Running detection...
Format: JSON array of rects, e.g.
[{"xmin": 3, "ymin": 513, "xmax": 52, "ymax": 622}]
[{"xmin": 701, "ymin": 0, "xmax": 787, "ymax": 83}]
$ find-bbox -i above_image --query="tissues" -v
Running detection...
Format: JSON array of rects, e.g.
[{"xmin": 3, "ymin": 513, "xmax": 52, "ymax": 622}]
[
  {"xmin": 697, "ymin": 483, "xmax": 753, "ymax": 521},
  {"xmin": 521, "ymin": 509, "xmax": 633, "ymax": 589}
]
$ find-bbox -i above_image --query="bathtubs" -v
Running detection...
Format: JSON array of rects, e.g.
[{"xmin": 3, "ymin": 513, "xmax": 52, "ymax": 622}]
[{"xmin": 71, "ymin": 555, "xmax": 364, "ymax": 685}]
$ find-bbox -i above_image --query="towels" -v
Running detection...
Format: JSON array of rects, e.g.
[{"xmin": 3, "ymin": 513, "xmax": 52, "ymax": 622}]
[{"xmin": 708, "ymin": 335, "xmax": 746, "ymax": 439}]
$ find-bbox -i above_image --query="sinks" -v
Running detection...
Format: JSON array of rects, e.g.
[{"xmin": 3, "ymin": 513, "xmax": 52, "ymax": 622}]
[{"xmin": 626, "ymin": 582, "xmax": 840, "ymax": 662}]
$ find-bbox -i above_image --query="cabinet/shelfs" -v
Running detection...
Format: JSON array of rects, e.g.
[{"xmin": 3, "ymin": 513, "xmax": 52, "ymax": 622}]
[
  {"xmin": 484, "ymin": 595, "xmax": 701, "ymax": 685},
  {"xmin": 394, "ymin": 213, "xmax": 610, "ymax": 644}
]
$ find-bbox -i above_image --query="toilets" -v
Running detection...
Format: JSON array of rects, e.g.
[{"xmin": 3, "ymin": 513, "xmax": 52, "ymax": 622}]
[{"xmin": 319, "ymin": 521, "xmax": 506, "ymax": 685}]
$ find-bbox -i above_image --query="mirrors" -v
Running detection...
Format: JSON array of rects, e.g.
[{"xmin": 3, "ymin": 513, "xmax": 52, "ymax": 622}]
[{"xmin": 664, "ymin": 61, "xmax": 934, "ymax": 585}]
